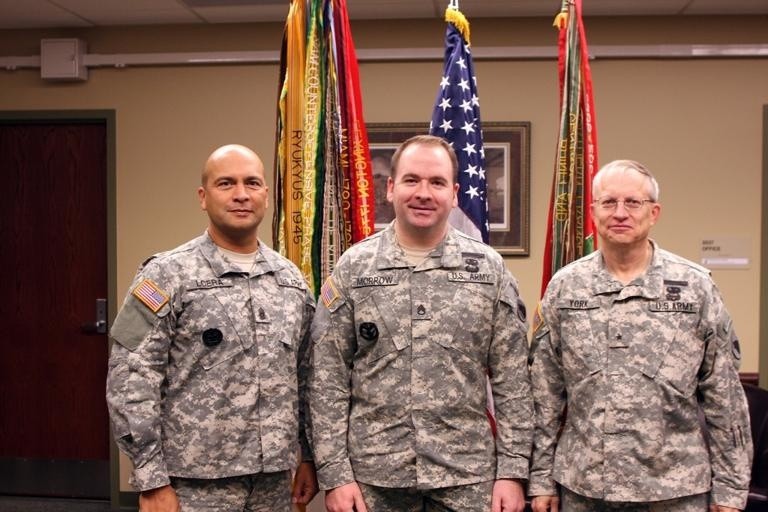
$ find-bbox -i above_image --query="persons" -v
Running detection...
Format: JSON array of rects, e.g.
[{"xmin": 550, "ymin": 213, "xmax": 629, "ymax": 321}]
[
  {"xmin": 301, "ymin": 134, "xmax": 535, "ymax": 512},
  {"xmin": 104, "ymin": 145, "xmax": 318, "ymax": 512},
  {"xmin": 525, "ymin": 158, "xmax": 753, "ymax": 512}
]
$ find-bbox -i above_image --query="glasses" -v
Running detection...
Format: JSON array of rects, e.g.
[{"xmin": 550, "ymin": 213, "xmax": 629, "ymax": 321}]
[{"xmin": 594, "ymin": 197, "xmax": 653, "ymax": 212}]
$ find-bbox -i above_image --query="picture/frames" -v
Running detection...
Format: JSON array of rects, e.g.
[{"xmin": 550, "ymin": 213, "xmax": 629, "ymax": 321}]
[{"xmin": 365, "ymin": 121, "xmax": 530, "ymax": 258}]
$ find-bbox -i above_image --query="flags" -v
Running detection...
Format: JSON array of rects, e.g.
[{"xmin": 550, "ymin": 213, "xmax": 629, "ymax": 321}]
[
  {"xmin": 537, "ymin": 0, "xmax": 601, "ymax": 301},
  {"xmin": 271, "ymin": 0, "xmax": 377, "ymax": 305},
  {"xmin": 427, "ymin": 4, "xmax": 492, "ymax": 245}
]
